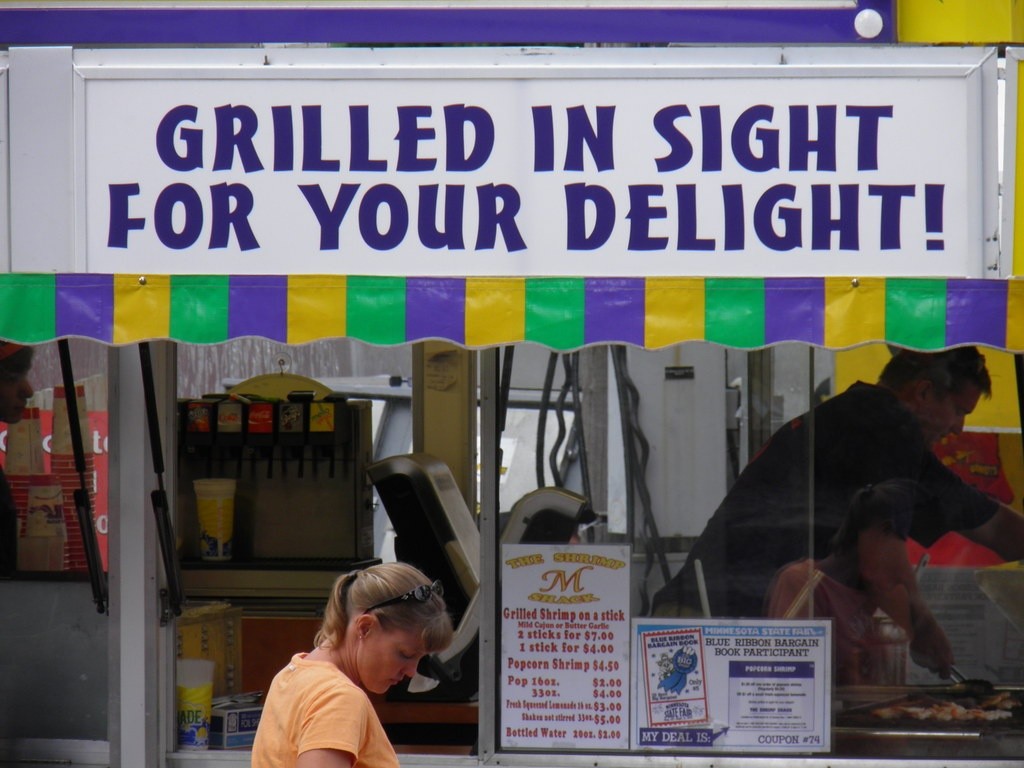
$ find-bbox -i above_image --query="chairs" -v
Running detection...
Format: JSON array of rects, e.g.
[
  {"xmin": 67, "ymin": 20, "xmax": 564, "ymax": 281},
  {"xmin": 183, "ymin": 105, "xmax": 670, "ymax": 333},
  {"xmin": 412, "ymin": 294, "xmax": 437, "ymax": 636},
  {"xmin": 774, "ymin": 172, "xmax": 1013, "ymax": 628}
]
[
  {"xmin": 428, "ymin": 489, "xmax": 599, "ymax": 679},
  {"xmin": 367, "ymin": 453, "xmax": 480, "ymax": 601}
]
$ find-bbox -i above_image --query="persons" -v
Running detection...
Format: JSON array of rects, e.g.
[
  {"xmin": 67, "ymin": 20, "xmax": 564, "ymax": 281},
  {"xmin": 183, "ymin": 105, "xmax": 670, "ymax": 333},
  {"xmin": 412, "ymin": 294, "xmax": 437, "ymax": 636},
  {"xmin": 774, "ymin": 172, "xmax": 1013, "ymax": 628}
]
[
  {"xmin": 766, "ymin": 478, "xmax": 951, "ymax": 718},
  {"xmin": 653, "ymin": 346, "xmax": 1024, "ymax": 679},
  {"xmin": 251, "ymin": 558, "xmax": 454, "ymax": 768},
  {"xmin": 0, "ymin": 339, "xmax": 34, "ymax": 570}
]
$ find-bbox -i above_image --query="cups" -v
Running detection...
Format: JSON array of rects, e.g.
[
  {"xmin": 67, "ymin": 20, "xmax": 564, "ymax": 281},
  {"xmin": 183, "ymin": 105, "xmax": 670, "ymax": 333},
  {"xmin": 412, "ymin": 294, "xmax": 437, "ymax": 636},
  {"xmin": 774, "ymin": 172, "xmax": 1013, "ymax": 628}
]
[
  {"xmin": 5, "ymin": 385, "xmax": 96, "ymax": 572},
  {"xmin": 194, "ymin": 479, "xmax": 236, "ymax": 559},
  {"xmin": 176, "ymin": 660, "xmax": 213, "ymax": 749}
]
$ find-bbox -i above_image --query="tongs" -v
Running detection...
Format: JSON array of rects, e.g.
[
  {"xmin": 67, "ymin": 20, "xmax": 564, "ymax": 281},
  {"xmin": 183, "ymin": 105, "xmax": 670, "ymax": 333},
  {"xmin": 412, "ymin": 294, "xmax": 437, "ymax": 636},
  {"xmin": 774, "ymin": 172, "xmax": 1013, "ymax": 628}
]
[
  {"xmin": 950, "ymin": 667, "xmax": 967, "ymax": 683},
  {"xmin": 842, "ymin": 679, "xmax": 993, "ymax": 697}
]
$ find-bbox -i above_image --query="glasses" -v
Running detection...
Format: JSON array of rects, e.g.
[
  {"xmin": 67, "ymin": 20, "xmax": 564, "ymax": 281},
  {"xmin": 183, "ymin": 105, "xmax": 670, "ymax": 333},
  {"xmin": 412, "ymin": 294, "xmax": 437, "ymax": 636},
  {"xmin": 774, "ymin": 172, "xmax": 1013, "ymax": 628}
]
[{"xmin": 361, "ymin": 579, "xmax": 445, "ymax": 617}]
